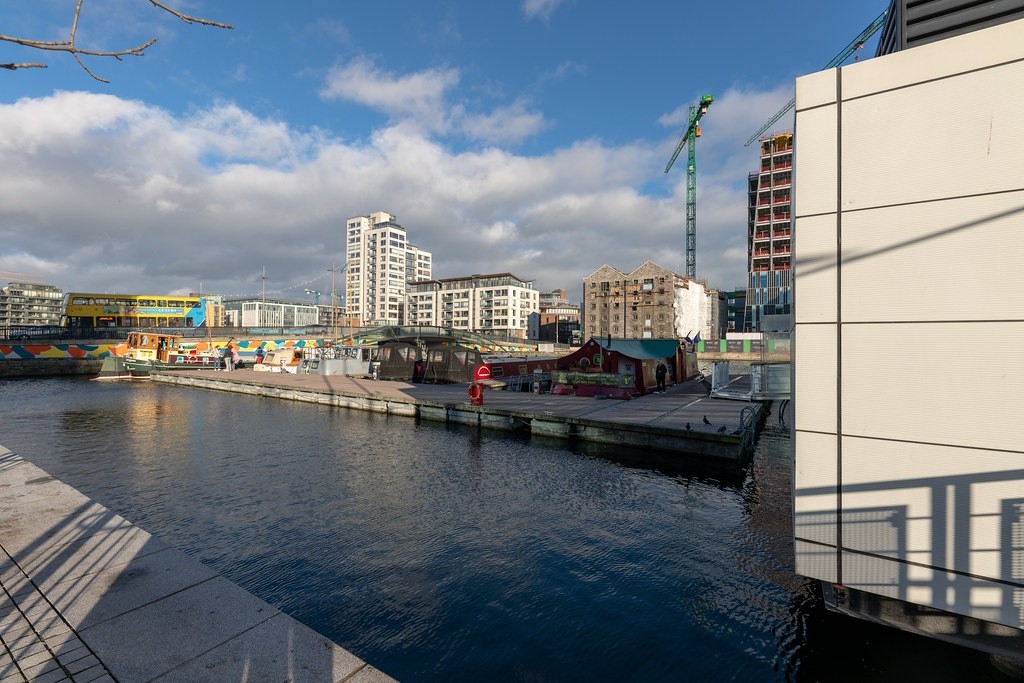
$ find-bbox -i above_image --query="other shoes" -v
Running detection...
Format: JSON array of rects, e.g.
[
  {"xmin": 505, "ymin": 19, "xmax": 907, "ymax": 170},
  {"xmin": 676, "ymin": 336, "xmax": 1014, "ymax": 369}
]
[
  {"xmin": 654, "ymin": 391, "xmax": 658, "ymax": 393},
  {"xmin": 662, "ymin": 392, "xmax": 666, "ymax": 394},
  {"xmin": 218, "ymin": 369, "xmax": 222, "ymax": 371},
  {"xmin": 224, "ymin": 369, "xmax": 231, "ymax": 371},
  {"xmin": 214, "ymin": 369, "xmax": 216, "ymax": 371}
]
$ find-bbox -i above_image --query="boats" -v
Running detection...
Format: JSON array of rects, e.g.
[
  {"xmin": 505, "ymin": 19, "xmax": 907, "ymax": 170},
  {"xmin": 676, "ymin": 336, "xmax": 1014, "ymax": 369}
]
[{"xmin": 89, "ymin": 329, "xmax": 230, "ymax": 383}]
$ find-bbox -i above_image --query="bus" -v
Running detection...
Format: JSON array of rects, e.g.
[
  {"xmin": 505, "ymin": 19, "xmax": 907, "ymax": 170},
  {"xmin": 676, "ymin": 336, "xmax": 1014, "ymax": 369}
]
[{"xmin": 57, "ymin": 292, "xmax": 209, "ymax": 340}]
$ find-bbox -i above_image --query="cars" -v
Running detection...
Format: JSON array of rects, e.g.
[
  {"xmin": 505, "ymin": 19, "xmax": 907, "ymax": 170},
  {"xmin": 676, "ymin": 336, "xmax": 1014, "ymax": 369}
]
[{"xmin": 9, "ymin": 323, "xmax": 64, "ymax": 341}]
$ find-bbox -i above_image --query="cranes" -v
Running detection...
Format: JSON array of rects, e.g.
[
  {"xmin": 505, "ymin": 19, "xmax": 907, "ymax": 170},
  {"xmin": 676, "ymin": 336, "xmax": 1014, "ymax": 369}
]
[{"xmin": 662, "ymin": 93, "xmax": 716, "ymax": 282}]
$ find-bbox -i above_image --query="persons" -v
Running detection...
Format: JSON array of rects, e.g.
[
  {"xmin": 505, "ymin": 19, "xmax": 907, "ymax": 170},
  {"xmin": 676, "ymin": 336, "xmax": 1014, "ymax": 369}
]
[
  {"xmin": 655, "ymin": 361, "xmax": 668, "ymax": 396},
  {"xmin": 209, "ymin": 345, "xmax": 264, "ymax": 371}
]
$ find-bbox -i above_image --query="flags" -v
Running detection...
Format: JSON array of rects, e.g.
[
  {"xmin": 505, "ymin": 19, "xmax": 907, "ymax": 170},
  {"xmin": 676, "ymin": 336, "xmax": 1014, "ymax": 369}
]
[{"xmin": 686, "ymin": 331, "xmax": 702, "ymax": 344}]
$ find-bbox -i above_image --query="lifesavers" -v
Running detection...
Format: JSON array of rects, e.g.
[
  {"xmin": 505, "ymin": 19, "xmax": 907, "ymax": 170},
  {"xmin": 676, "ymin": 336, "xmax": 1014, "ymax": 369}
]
[{"xmin": 468, "ymin": 383, "xmax": 483, "ymax": 401}]
